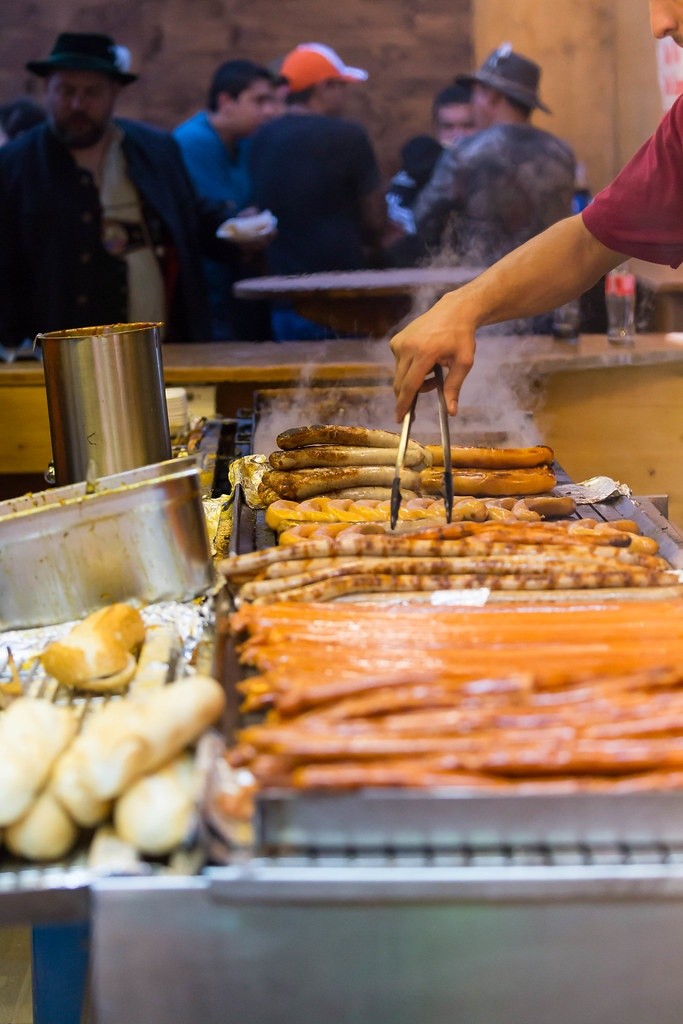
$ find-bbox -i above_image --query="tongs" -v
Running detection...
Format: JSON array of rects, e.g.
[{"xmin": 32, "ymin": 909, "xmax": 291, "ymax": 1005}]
[{"xmin": 389, "ymin": 363, "xmax": 454, "ymax": 531}]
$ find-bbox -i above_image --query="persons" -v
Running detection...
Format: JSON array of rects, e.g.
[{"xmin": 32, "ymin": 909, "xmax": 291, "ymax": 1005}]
[
  {"xmin": 173, "ymin": 60, "xmax": 337, "ymax": 342},
  {"xmin": 384, "ymin": 54, "xmax": 580, "ymax": 338},
  {"xmin": 243, "ymin": 40, "xmax": 387, "ymax": 273},
  {"xmin": 378, "ymin": 87, "xmax": 480, "ymax": 238},
  {"xmin": 0, "ymin": 100, "xmax": 48, "ymax": 143},
  {"xmin": 0, "ymin": 30, "xmax": 278, "ymax": 345},
  {"xmin": 389, "ymin": 1, "xmax": 682, "ymax": 423}
]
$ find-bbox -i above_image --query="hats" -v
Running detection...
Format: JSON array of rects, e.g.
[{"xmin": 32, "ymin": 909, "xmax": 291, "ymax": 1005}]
[
  {"xmin": 25, "ymin": 32, "xmax": 139, "ymax": 88},
  {"xmin": 455, "ymin": 46, "xmax": 551, "ymax": 114},
  {"xmin": 279, "ymin": 42, "xmax": 368, "ymax": 92}
]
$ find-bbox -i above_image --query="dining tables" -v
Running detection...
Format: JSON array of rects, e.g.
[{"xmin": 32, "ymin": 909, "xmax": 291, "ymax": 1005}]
[{"xmin": 234, "ymin": 266, "xmax": 490, "ymax": 338}]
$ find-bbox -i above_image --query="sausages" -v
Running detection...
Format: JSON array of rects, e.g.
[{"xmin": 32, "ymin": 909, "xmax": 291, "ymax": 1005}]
[
  {"xmin": 257, "ymin": 425, "xmax": 658, "ymax": 554},
  {"xmin": 0, "ymin": 679, "xmax": 224, "ymax": 858}
]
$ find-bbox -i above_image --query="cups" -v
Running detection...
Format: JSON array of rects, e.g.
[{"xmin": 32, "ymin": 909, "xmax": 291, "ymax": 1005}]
[{"xmin": 551, "ymin": 294, "xmax": 580, "ymax": 346}]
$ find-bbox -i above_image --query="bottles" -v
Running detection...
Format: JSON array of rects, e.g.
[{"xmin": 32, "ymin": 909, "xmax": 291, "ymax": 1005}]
[{"xmin": 604, "ymin": 261, "xmax": 637, "ymax": 346}]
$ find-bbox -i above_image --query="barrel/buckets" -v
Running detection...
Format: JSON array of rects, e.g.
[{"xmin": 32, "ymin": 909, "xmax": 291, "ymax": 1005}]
[{"xmin": 34, "ymin": 320, "xmax": 173, "ymax": 488}]
